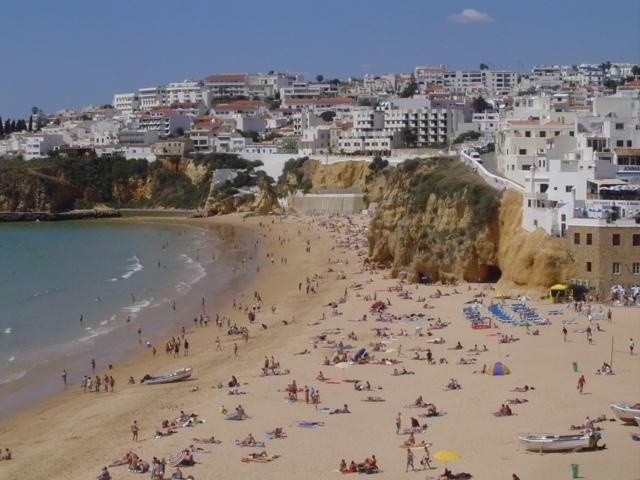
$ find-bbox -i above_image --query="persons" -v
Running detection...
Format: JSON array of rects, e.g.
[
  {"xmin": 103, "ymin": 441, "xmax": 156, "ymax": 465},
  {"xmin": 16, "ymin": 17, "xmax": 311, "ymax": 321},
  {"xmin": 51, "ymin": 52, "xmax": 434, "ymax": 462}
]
[
  {"xmin": 77, "ymin": 275, "xmax": 640, "ymax": 479},
  {"xmin": 152, "ymin": 211, "xmax": 379, "ymax": 274},
  {"xmin": 1, "ymin": 447, "xmax": 13, "ymax": 461},
  {"xmin": 61, "ymin": 369, "xmax": 68, "ymax": 385}
]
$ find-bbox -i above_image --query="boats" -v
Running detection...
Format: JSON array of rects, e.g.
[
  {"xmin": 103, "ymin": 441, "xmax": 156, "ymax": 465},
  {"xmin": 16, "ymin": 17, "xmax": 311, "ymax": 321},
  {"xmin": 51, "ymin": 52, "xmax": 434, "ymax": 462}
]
[
  {"xmin": 139, "ymin": 364, "xmax": 194, "ymax": 385},
  {"xmin": 517, "ymin": 428, "xmax": 600, "ymax": 454},
  {"xmin": 609, "ymin": 402, "xmax": 640, "ymax": 442}
]
